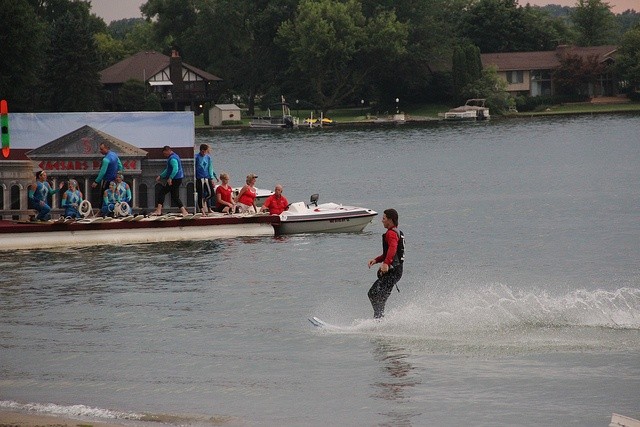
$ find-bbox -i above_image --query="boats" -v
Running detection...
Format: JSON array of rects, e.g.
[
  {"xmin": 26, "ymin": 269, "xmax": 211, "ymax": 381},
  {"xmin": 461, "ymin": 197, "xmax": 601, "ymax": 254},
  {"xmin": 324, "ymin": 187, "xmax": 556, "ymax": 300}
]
[
  {"xmin": 0, "ymin": 101, "xmax": 285, "ymax": 252},
  {"xmin": 213, "ymin": 183, "xmax": 275, "ymax": 206},
  {"xmin": 249, "ymin": 95, "xmax": 300, "ymax": 129},
  {"xmin": 444, "ymin": 98, "xmax": 491, "ymax": 120},
  {"xmin": 274, "ymin": 185, "xmax": 379, "ymax": 235}
]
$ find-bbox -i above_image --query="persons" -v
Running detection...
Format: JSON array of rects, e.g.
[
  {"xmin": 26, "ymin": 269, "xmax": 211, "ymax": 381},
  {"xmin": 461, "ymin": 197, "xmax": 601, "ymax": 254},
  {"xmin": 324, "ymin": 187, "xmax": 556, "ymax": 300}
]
[
  {"xmin": 216, "ymin": 173, "xmax": 236, "ymax": 214},
  {"xmin": 28, "ymin": 170, "xmax": 64, "ymax": 222},
  {"xmin": 204, "ymin": 145, "xmax": 220, "ymax": 214},
  {"xmin": 194, "ymin": 144, "xmax": 213, "ymax": 215},
  {"xmin": 233, "ymin": 173, "xmax": 258, "ymax": 214},
  {"xmin": 259, "ymin": 184, "xmax": 289, "ymax": 215},
  {"xmin": 114, "ymin": 171, "xmax": 132, "ymax": 203},
  {"xmin": 102, "ymin": 181, "xmax": 119, "ymax": 217},
  {"xmin": 149, "ymin": 145, "xmax": 189, "ymax": 217},
  {"xmin": 62, "ymin": 178, "xmax": 84, "ymax": 219},
  {"xmin": 90, "ymin": 141, "xmax": 123, "ymax": 213},
  {"xmin": 366, "ymin": 208, "xmax": 406, "ymax": 316}
]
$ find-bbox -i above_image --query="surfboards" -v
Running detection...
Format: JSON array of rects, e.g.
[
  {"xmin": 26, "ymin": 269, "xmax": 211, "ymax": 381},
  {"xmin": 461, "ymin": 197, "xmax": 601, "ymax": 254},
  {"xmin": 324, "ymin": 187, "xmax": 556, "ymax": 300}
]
[
  {"xmin": 308, "ymin": 318, "xmax": 319, "ymax": 326},
  {"xmin": 313, "ymin": 316, "xmax": 324, "ymax": 324}
]
